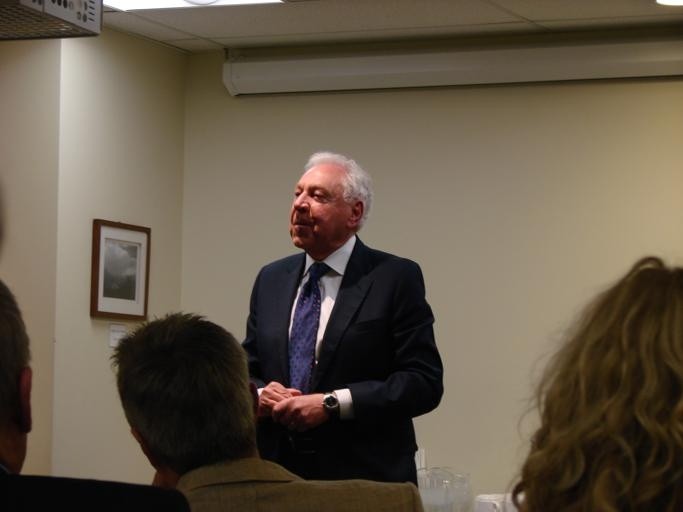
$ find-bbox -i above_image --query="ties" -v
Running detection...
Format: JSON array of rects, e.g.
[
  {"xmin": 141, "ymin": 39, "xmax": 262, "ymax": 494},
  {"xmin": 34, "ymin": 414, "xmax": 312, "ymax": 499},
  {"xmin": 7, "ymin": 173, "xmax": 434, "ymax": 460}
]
[{"xmin": 288, "ymin": 263, "xmax": 332, "ymax": 394}]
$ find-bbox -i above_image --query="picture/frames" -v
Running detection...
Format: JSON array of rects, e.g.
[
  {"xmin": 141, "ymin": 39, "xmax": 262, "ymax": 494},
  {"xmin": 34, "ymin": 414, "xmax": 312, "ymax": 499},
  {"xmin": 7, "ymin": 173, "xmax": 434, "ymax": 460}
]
[{"xmin": 89, "ymin": 216, "xmax": 152, "ymax": 323}]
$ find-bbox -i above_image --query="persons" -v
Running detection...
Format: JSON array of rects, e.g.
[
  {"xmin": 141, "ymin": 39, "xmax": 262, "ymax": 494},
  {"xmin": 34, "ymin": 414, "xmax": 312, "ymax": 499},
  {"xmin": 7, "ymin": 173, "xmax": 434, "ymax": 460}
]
[
  {"xmin": 238, "ymin": 150, "xmax": 442, "ymax": 488},
  {"xmin": 109, "ymin": 309, "xmax": 425, "ymax": 511},
  {"xmin": 0, "ymin": 280, "xmax": 187, "ymax": 507},
  {"xmin": 498, "ymin": 257, "xmax": 681, "ymax": 512}
]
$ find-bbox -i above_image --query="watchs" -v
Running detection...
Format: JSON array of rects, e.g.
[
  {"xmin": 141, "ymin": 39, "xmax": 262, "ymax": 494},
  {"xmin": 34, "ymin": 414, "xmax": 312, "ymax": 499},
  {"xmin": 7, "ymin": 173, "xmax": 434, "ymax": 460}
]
[{"xmin": 320, "ymin": 392, "xmax": 339, "ymax": 418}]
